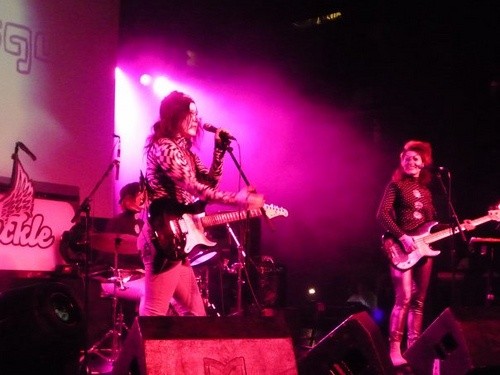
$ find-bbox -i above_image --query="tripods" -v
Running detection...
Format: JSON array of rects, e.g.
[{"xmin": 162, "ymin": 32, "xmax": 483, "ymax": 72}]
[{"xmin": 78, "ymin": 240, "xmax": 130, "ymax": 368}]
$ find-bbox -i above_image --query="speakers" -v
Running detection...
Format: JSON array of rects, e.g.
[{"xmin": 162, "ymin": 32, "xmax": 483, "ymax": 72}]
[
  {"xmin": 0, "ymin": 284, "xmax": 83, "ymax": 375},
  {"xmin": 0, "ymin": 175, "xmax": 81, "ymax": 284},
  {"xmin": 296, "ymin": 310, "xmax": 398, "ymax": 375},
  {"xmin": 401, "ymin": 307, "xmax": 473, "ymax": 375},
  {"xmin": 132, "ymin": 314, "xmax": 298, "ymax": 375}
]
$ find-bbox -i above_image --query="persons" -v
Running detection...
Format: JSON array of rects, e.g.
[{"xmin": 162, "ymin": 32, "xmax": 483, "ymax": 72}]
[
  {"xmin": 317, "ymin": 272, "xmax": 384, "ymax": 337},
  {"xmin": 119, "ymin": 182, "xmax": 149, "ymax": 235},
  {"xmin": 108, "ymin": 91, "xmax": 264, "ymax": 375},
  {"xmin": 377, "ymin": 139, "xmax": 474, "ymax": 368}
]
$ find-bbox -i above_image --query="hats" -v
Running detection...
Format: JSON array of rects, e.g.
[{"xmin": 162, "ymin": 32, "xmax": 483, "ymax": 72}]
[{"xmin": 118, "ymin": 181, "xmax": 143, "ymax": 205}]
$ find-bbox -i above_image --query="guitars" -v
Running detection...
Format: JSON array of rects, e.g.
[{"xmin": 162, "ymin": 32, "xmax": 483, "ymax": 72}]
[
  {"xmin": 148, "ymin": 199, "xmax": 289, "ymax": 262},
  {"xmin": 381, "ymin": 209, "xmax": 500, "ymax": 271}
]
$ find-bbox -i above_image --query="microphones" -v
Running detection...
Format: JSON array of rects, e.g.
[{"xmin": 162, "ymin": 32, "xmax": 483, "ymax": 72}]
[
  {"xmin": 202, "ymin": 123, "xmax": 237, "ymax": 141},
  {"xmin": 418, "ymin": 163, "xmax": 443, "ymax": 170},
  {"xmin": 17, "ymin": 141, "xmax": 37, "ymax": 161},
  {"xmin": 116, "ymin": 137, "xmax": 120, "ymax": 158}
]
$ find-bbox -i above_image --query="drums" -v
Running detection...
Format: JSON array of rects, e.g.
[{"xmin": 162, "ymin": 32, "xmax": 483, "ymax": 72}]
[{"xmin": 189, "ymin": 244, "xmax": 217, "ymax": 266}]
